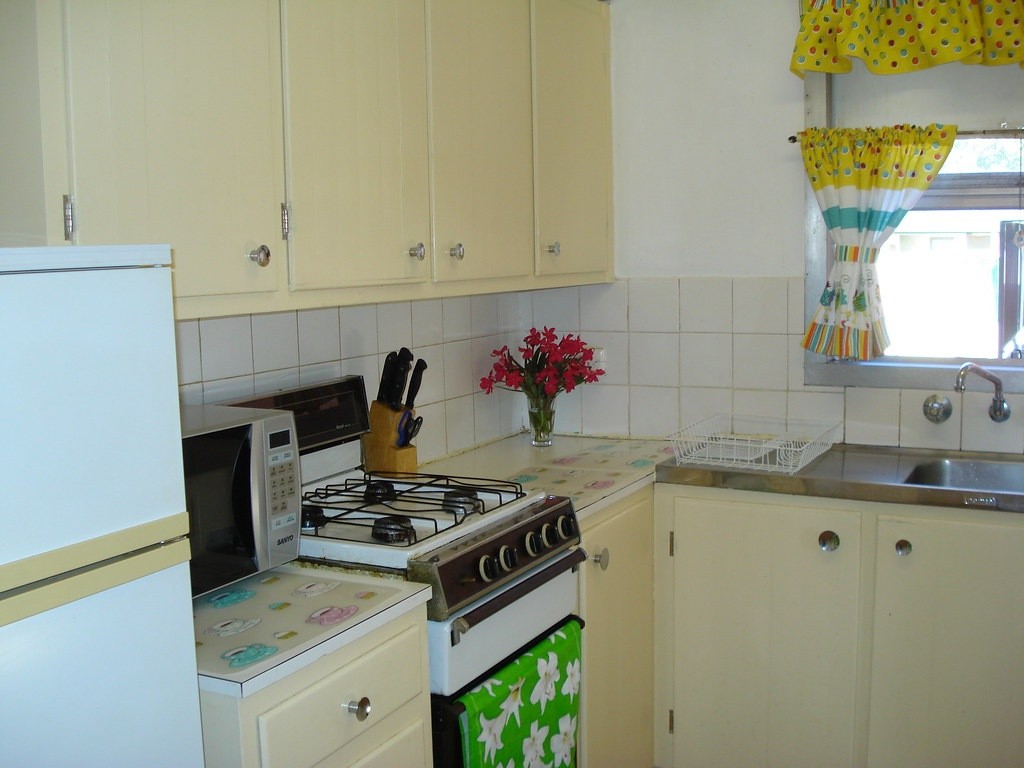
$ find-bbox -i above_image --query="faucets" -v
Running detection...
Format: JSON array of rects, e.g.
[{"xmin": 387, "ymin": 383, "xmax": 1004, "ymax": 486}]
[{"xmin": 952, "ymin": 362, "xmax": 1012, "ymax": 425}]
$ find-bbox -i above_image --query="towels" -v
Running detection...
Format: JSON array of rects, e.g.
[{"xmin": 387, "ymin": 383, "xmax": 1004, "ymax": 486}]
[{"xmin": 452, "ymin": 618, "xmax": 582, "ymax": 768}]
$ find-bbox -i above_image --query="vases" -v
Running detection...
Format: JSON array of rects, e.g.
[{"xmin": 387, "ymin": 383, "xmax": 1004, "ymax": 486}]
[{"xmin": 525, "ymin": 395, "xmax": 558, "ymax": 446}]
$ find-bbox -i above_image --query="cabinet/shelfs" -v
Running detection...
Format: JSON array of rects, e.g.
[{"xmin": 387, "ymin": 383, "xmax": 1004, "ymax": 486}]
[
  {"xmin": 651, "ymin": 482, "xmax": 1024, "ymax": 768},
  {"xmin": 0, "ymin": 0, "xmax": 616, "ymax": 319},
  {"xmin": 201, "ymin": 600, "xmax": 434, "ymax": 768},
  {"xmin": 578, "ymin": 485, "xmax": 655, "ymax": 768}
]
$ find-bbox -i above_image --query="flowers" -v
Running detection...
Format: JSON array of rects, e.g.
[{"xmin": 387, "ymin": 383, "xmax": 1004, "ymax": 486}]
[{"xmin": 479, "ymin": 326, "xmax": 606, "ymax": 439}]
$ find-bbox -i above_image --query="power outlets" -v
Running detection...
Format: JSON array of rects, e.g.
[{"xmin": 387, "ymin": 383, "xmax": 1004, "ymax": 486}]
[{"xmin": 586, "ymin": 348, "xmax": 606, "ymax": 370}]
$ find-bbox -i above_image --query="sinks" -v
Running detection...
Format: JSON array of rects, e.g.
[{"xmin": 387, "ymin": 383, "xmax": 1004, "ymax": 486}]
[{"xmin": 901, "ymin": 459, "xmax": 1024, "ymax": 496}]
